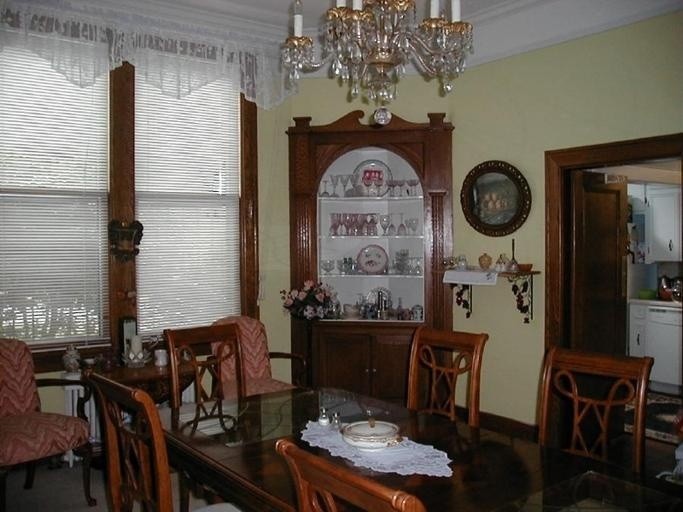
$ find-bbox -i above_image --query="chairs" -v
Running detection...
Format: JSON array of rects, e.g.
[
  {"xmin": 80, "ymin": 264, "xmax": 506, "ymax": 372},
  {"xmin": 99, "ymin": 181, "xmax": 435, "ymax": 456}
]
[
  {"xmin": 536, "ymin": 348, "xmax": 655, "ymax": 475},
  {"xmin": 164, "ymin": 317, "xmax": 248, "ymax": 511},
  {"xmin": 276, "ymin": 439, "xmax": 424, "ymax": 511},
  {"xmin": 87, "ymin": 371, "xmax": 174, "ymax": 512},
  {"xmin": 408, "ymin": 324, "xmax": 489, "ymax": 429},
  {"xmin": 0, "ymin": 338, "xmax": 100, "ymax": 507},
  {"xmin": 212, "ymin": 317, "xmax": 296, "ymax": 401}
]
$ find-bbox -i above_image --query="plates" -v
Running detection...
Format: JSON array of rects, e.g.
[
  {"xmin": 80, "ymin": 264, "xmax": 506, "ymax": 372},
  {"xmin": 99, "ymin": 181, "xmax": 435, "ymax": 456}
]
[{"xmin": 351, "ymin": 159, "xmax": 392, "ymax": 196}]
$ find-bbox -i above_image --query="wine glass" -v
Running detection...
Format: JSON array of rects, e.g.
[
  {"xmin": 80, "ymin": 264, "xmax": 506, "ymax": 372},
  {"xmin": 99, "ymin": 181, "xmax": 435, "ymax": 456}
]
[{"xmin": 317, "ymin": 173, "xmax": 423, "ymax": 275}]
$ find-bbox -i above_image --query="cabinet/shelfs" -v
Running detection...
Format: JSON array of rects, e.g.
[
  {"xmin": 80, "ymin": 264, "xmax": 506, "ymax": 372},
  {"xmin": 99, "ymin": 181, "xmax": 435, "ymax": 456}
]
[
  {"xmin": 286, "ymin": 106, "xmax": 455, "ymax": 416},
  {"xmin": 642, "ymin": 182, "xmax": 682, "ymax": 268},
  {"xmin": 628, "ymin": 298, "xmax": 681, "ymax": 397}
]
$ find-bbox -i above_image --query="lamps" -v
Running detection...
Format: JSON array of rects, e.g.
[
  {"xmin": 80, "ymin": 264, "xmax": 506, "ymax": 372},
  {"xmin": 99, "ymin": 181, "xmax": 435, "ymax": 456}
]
[{"xmin": 275, "ymin": 1, "xmax": 474, "ymax": 126}]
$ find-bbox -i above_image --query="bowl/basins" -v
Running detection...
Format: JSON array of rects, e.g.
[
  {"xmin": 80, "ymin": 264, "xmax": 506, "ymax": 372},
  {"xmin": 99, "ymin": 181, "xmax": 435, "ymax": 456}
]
[
  {"xmin": 342, "ymin": 420, "xmax": 399, "ymax": 452},
  {"xmin": 637, "ymin": 289, "xmax": 656, "ymax": 299}
]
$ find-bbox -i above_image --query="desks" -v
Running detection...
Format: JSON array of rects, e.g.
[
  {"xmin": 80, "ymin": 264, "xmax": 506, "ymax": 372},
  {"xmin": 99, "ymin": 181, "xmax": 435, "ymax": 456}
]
[{"xmin": 97, "ymin": 365, "xmax": 200, "ymax": 406}]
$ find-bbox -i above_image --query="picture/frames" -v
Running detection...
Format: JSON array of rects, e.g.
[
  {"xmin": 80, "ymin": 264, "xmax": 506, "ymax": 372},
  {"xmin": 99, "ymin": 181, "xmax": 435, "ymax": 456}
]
[{"xmin": 460, "ymin": 159, "xmax": 533, "ymax": 238}]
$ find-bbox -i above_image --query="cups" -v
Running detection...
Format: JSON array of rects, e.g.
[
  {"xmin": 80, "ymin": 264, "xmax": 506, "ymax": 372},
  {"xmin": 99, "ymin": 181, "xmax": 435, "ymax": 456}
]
[{"xmin": 154, "ymin": 348, "xmax": 191, "ymax": 367}]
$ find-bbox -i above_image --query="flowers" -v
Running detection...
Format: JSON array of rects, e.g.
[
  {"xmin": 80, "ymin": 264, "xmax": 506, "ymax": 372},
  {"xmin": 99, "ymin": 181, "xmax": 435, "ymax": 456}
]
[{"xmin": 277, "ymin": 276, "xmax": 340, "ymax": 320}]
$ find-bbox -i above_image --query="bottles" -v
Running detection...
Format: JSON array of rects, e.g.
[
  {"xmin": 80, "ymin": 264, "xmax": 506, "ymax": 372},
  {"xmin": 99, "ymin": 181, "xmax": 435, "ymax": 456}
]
[
  {"xmin": 62, "ymin": 344, "xmax": 112, "ymax": 375},
  {"xmin": 658, "ymin": 272, "xmax": 682, "ymax": 302},
  {"xmin": 318, "ymin": 407, "xmax": 330, "ymax": 426},
  {"xmin": 340, "ymin": 286, "xmax": 422, "ymax": 321},
  {"xmin": 330, "ymin": 411, "xmax": 342, "ymax": 430},
  {"xmin": 440, "ymin": 254, "xmax": 533, "ymax": 270}
]
